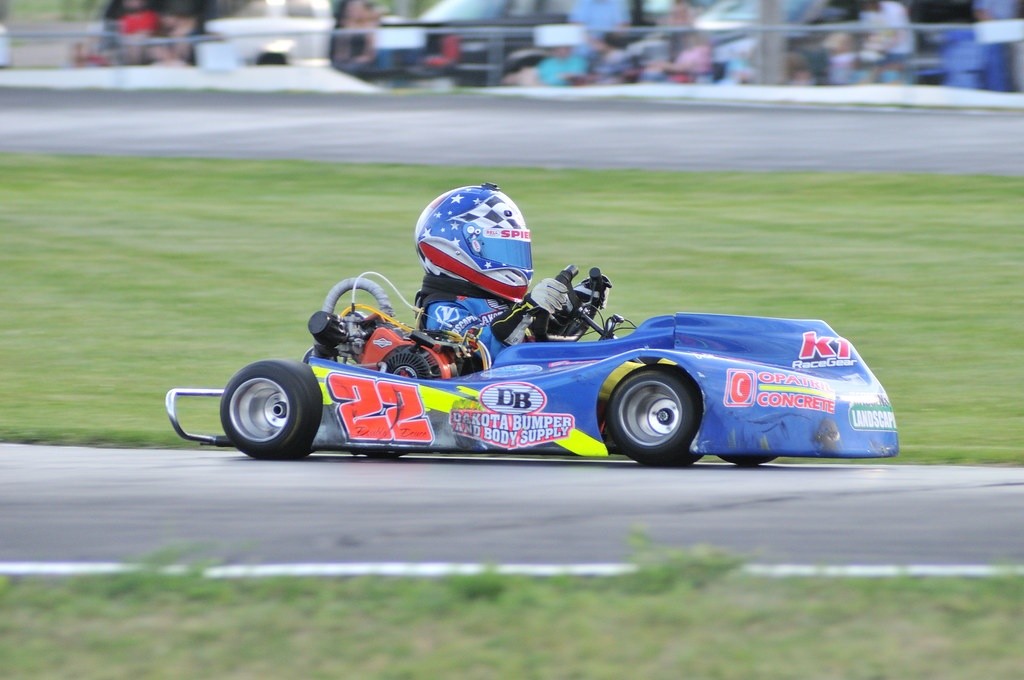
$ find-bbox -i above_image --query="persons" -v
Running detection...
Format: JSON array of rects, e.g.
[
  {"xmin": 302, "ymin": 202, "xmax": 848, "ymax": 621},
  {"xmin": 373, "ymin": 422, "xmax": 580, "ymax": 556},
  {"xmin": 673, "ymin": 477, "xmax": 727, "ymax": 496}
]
[
  {"xmin": 415, "ymin": 183, "xmax": 609, "ymax": 377},
  {"xmin": 80, "ymin": 0, "xmax": 1024, "ymax": 95}
]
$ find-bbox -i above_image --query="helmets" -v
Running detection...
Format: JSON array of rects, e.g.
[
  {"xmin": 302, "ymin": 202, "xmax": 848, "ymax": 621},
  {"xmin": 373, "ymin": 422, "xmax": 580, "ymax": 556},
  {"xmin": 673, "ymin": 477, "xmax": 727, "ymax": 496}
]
[{"xmin": 415, "ymin": 182, "xmax": 534, "ymax": 303}]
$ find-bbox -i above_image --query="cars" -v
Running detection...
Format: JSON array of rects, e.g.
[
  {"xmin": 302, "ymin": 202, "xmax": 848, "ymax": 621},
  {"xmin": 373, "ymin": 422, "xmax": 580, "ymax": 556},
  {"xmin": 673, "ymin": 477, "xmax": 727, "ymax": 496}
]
[
  {"xmin": 381, "ymin": 0, "xmax": 569, "ymax": 85},
  {"xmin": 204, "ymin": 1, "xmax": 334, "ymax": 65}
]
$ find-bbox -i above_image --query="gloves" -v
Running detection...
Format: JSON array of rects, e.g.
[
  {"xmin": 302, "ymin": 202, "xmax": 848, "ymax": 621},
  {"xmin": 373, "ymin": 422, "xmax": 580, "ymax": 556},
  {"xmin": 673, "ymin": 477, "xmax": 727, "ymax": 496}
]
[
  {"xmin": 490, "ymin": 277, "xmax": 569, "ymax": 346},
  {"xmin": 573, "ymin": 274, "xmax": 612, "ymax": 310}
]
